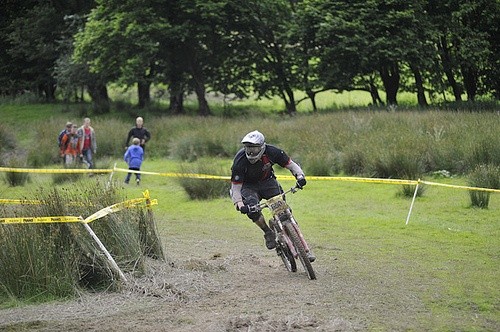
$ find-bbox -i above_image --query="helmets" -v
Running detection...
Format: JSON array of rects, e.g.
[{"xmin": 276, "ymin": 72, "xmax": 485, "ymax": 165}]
[{"xmin": 241, "ymin": 130, "xmax": 266, "ymax": 164}]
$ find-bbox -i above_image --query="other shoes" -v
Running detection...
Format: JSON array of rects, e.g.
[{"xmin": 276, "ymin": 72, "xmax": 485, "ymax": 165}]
[
  {"xmin": 307, "ymin": 253, "xmax": 316, "ymax": 262},
  {"xmin": 264, "ymin": 231, "xmax": 276, "ymax": 250}
]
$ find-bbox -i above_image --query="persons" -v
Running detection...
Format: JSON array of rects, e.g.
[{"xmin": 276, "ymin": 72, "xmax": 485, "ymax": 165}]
[
  {"xmin": 230, "ymin": 130, "xmax": 316, "ymax": 262},
  {"xmin": 58, "ymin": 117, "xmax": 151, "ymax": 186}
]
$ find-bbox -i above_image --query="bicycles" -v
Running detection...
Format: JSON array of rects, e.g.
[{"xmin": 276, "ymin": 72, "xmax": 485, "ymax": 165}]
[{"xmin": 249, "ymin": 182, "xmax": 317, "ymax": 280}]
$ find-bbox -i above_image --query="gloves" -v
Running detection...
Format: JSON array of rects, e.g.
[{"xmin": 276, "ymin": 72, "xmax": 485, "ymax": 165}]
[
  {"xmin": 295, "ymin": 178, "xmax": 306, "ymax": 190},
  {"xmin": 240, "ymin": 205, "xmax": 249, "ymax": 214}
]
